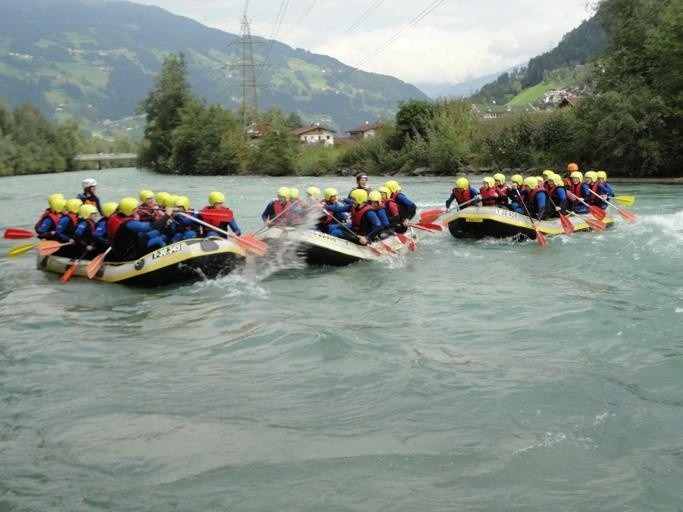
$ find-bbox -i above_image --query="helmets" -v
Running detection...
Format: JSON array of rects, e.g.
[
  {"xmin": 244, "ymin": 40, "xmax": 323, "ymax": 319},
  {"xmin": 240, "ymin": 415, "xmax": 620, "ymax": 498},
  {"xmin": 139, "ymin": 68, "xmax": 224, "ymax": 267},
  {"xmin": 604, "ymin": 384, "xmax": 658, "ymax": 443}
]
[
  {"xmin": 323, "ymin": 188, "xmax": 338, "ymax": 201},
  {"xmin": 483, "ymin": 173, "xmax": 522, "ymax": 188},
  {"xmin": 567, "ymin": 163, "xmax": 608, "ymax": 183},
  {"xmin": 357, "ymin": 172, "xmax": 369, "ymax": 181},
  {"xmin": 48, "ymin": 193, "xmax": 98, "ymax": 219},
  {"xmin": 118, "ymin": 197, "xmax": 138, "ymax": 217},
  {"xmin": 454, "ymin": 178, "xmax": 468, "ymax": 190},
  {"xmin": 102, "ymin": 203, "xmax": 118, "ymax": 217},
  {"xmin": 524, "ymin": 170, "xmax": 565, "ymax": 189},
  {"xmin": 140, "ymin": 190, "xmax": 189, "ymax": 211},
  {"xmin": 208, "ymin": 192, "xmax": 224, "ymax": 206},
  {"xmin": 82, "ymin": 178, "xmax": 98, "ymax": 188},
  {"xmin": 350, "ymin": 180, "xmax": 400, "ymax": 207},
  {"xmin": 278, "ymin": 187, "xmax": 320, "ymax": 201}
]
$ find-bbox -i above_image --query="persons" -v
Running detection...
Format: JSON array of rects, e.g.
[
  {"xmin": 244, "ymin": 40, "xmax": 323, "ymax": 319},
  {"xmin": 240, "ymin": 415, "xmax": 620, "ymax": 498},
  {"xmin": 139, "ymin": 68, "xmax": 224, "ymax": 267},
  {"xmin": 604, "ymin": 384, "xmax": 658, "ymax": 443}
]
[
  {"xmin": 444, "ymin": 163, "xmax": 616, "ymax": 228},
  {"xmin": 32, "ymin": 178, "xmax": 240, "ymax": 267},
  {"xmin": 260, "ymin": 172, "xmax": 417, "ymax": 247}
]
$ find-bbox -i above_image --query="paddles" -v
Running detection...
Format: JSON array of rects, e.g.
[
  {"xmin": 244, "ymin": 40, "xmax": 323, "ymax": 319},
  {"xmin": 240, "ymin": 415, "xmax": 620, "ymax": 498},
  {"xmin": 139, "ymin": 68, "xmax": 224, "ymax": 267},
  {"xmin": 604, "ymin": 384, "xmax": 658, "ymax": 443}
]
[
  {"xmin": 515, "ymin": 188, "xmax": 639, "ymax": 245},
  {"xmin": 320, "ymin": 196, "xmax": 477, "ymax": 256},
  {"xmin": 177, "ymin": 211, "xmax": 267, "ymax": 256},
  {"xmin": 4, "ymin": 228, "xmax": 112, "ymax": 284},
  {"xmin": 236, "ymin": 201, "xmax": 299, "ymax": 248}
]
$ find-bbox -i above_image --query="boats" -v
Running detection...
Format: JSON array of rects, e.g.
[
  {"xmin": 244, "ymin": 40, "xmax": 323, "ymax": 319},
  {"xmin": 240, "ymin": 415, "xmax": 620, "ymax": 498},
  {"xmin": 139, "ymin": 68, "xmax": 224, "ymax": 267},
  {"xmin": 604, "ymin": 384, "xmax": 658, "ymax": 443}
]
[
  {"xmin": 253, "ymin": 230, "xmax": 420, "ymax": 269},
  {"xmin": 446, "ymin": 206, "xmax": 608, "ymax": 239},
  {"xmin": 37, "ymin": 237, "xmax": 248, "ymax": 288}
]
